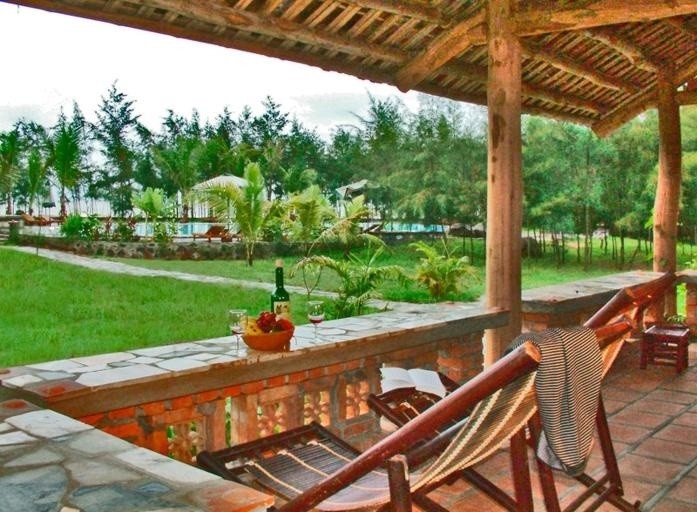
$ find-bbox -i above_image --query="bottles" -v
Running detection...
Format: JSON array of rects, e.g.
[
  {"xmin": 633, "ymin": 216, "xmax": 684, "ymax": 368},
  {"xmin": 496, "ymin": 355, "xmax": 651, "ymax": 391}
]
[{"xmin": 271, "ymin": 259, "xmax": 290, "ymax": 317}]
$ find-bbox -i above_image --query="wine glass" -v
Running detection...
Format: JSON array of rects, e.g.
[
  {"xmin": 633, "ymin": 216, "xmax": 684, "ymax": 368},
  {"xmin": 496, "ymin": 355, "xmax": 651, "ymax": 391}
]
[
  {"xmin": 228, "ymin": 309, "xmax": 248, "ymax": 359},
  {"xmin": 307, "ymin": 301, "xmax": 326, "ymax": 345}
]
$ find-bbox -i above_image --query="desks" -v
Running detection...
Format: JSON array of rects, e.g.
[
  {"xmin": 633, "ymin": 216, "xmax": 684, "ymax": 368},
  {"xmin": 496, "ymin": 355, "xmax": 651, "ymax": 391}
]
[{"xmin": 221, "ymin": 229, "xmax": 232, "ymax": 242}]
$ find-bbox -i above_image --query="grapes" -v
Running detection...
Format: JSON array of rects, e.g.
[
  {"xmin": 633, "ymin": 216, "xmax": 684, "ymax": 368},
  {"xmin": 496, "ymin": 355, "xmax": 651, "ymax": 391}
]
[{"xmin": 256, "ymin": 312, "xmax": 278, "ymax": 334}]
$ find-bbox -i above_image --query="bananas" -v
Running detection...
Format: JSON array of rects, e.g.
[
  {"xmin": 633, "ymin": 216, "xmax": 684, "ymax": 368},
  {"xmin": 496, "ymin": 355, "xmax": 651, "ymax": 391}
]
[{"xmin": 239, "ymin": 317, "xmax": 263, "ymax": 334}]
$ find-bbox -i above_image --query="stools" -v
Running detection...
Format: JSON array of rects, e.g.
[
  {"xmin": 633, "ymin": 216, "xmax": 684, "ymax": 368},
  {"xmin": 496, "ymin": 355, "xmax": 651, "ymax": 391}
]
[{"xmin": 640, "ymin": 324, "xmax": 690, "ymax": 374}]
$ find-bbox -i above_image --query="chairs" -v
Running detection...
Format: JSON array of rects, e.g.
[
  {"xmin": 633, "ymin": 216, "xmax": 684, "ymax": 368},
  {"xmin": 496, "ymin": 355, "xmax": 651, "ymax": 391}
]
[
  {"xmin": 232, "ymin": 229, "xmax": 244, "ymax": 242},
  {"xmin": 191, "ymin": 224, "xmax": 226, "ymax": 242},
  {"xmin": 21, "ymin": 213, "xmax": 40, "ymax": 226},
  {"xmin": 363, "ymin": 223, "xmax": 385, "ymax": 233},
  {"xmin": 197, "ymin": 321, "xmax": 633, "ymax": 511},
  {"xmin": 40, "ymin": 215, "xmax": 62, "ymax": 227},
  {"xmin": 366, "ymin": 272, "xmax": 689, "ymax": 511}
]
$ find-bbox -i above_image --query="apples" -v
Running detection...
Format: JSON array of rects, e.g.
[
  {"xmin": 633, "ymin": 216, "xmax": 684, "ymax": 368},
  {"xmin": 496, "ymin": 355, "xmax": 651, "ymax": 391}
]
[{"xmin": 273, "ymin": 318, "xmax": 293, "ymax": 334}]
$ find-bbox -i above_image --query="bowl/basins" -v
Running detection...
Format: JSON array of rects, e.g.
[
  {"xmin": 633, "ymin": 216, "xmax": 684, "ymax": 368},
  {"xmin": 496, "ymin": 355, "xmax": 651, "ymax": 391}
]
[{"xmin": 239, "ymin": 326, "xmax": 296, "ymax": 351}]
